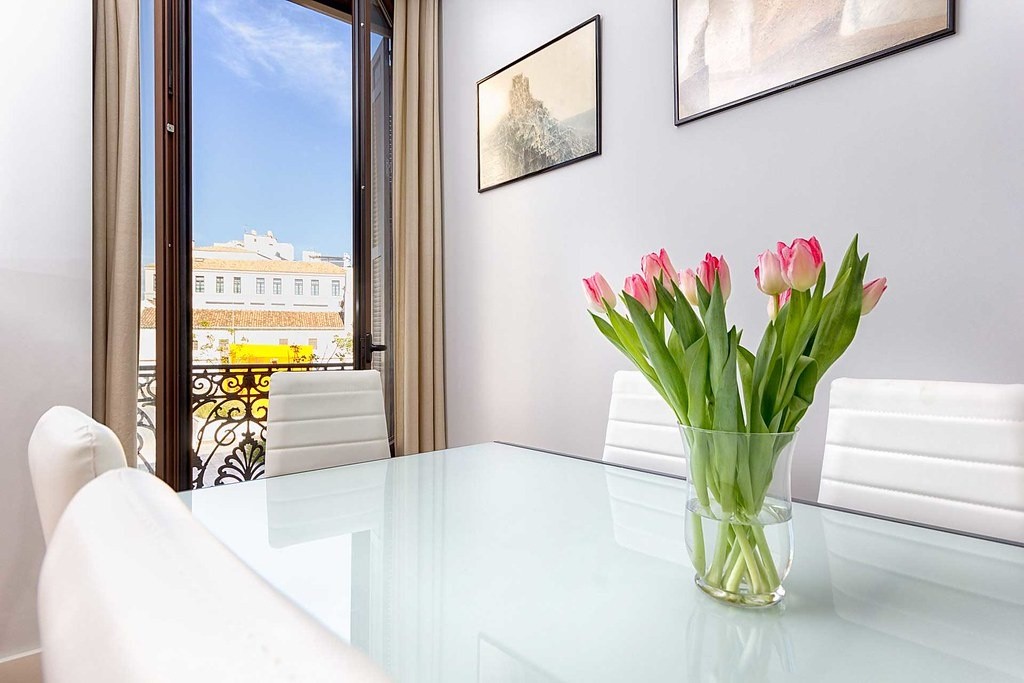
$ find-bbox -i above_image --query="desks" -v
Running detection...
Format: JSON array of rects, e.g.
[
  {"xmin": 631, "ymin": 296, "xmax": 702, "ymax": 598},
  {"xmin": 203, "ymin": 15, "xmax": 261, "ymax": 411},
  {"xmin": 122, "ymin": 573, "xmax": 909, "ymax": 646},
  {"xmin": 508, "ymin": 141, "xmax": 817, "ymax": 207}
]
[{"xmin": 175, "ymin": 442, "xmax": 1023, "ymax": 682}]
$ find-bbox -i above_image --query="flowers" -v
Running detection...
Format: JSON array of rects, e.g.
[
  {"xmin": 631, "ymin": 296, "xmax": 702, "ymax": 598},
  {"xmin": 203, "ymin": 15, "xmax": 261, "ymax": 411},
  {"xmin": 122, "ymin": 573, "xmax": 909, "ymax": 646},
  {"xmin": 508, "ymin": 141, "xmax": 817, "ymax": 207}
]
[{"xmin": 583, "ymin": 234, "xmax": 887, "ymax": 605}]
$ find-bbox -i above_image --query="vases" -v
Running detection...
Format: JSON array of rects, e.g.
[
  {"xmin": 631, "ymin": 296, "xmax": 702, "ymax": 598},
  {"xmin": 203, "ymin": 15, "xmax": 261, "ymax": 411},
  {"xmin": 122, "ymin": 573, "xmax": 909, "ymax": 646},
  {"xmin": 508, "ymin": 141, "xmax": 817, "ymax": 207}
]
[{"xmin": 677, "ymin": 422, "xmax": 800, "ymax": 610}]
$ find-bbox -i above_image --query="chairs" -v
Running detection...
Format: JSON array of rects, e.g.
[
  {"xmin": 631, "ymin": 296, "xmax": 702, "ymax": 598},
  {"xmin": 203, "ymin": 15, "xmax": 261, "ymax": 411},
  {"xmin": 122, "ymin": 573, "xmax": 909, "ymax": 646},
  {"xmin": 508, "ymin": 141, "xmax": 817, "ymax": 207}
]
[
  {"xmin": 265, "ymin": 370, "xmax": 392, "ymax": 478},
  {"xmin": 28, "ymin": 406, "xmax": 128, "ymax": 550},
  {"xmin": 602, "ymin": 370, "xmax": 749, "ymax": 480},
  {"xmin": 817, "ymin": 378, "xmax": 1024, "ymax": 545},
  {"xmin": 35, "ymin": 466, "xmax": 390, "ymax": 683}
]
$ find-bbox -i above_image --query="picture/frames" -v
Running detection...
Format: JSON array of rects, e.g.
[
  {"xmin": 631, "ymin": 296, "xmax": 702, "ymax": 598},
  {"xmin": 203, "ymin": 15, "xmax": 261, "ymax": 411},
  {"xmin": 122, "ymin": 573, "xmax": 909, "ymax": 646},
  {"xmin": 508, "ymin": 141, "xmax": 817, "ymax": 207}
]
[
  {"xmin": 476, "ymin": 15, "xmax": 601, "ymax": 193},
  {"xmin": 673, "ymin": 0, "xmax": 956, "ymax": 126}
]
